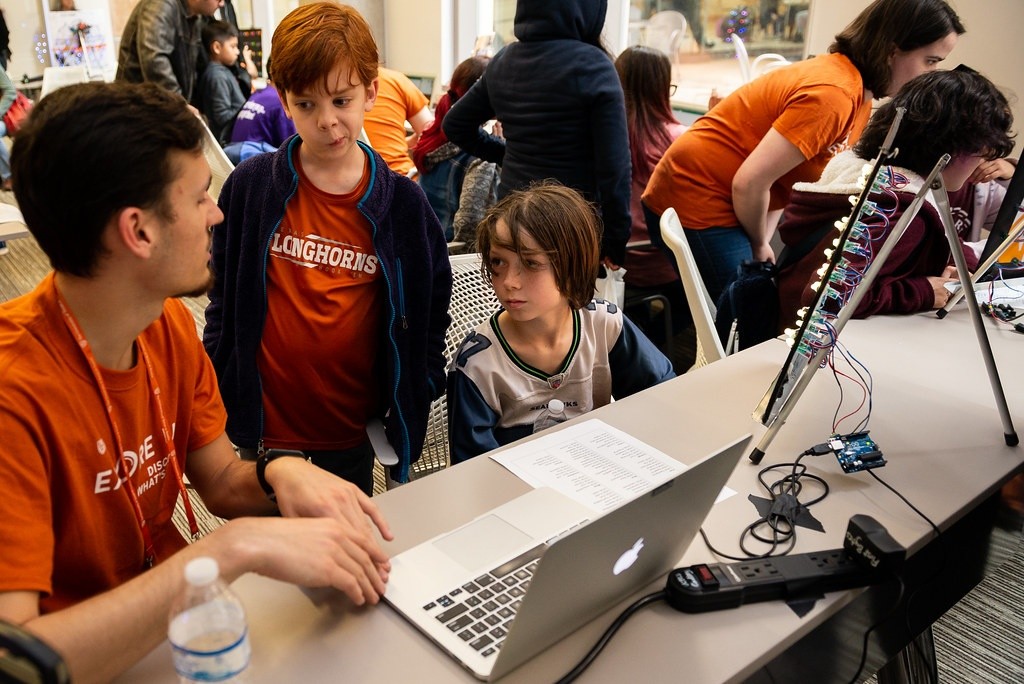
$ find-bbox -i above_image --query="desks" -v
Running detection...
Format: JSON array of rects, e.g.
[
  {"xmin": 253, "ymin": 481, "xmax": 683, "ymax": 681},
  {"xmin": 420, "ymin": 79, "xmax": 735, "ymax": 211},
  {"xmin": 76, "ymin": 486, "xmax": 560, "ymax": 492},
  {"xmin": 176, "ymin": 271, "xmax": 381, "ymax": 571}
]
[{"xmin": 113, "ymin": 274, "xmax": 1023, "ymax": 683}]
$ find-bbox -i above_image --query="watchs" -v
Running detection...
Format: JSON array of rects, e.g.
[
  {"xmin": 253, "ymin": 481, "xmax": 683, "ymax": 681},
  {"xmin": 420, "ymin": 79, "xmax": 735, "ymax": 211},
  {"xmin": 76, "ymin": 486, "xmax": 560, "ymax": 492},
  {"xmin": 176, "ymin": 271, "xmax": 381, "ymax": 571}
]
[{"xmin": 255, "ymin": 448, "xmax": 307, "ymax": 498}]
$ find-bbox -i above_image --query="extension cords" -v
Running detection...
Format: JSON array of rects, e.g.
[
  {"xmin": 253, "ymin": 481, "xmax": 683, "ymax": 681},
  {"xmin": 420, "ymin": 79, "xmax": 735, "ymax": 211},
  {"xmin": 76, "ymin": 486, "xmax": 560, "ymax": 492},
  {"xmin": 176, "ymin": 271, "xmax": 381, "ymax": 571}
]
[{"xmin": 665, "ymin": 547, "xmax": 895, "ymax": 614}]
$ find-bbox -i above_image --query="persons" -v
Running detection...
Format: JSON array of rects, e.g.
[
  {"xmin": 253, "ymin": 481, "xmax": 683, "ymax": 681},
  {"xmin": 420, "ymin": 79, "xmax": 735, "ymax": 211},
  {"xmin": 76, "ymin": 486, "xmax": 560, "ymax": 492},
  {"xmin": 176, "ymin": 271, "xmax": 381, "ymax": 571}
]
[
  {"xmin": 359, "ymin": 63, "xmax": 435, "ymax": 203},
  {"xmin": 768, "ymin": 70, "xmax": 1019, "ymax": 336},
  {"xmin": 0, "ymin": 80, "xmax": 396, "ymax": 684},
  {"xmin": 51, "ymin": 0, "xmax": 78, "ymax": 11},
  {"xmin": 444, "ymin": 184, "xmax": 676, "ymax": 474},
  {"xmin": 611, "ymin": 46, "xmax": 686, "ymax": 295},
  {"xmin": 113, "ymin": 0, "xmax": 226, "ymax": 130},
  {"xmin": 639, "ymin": 0, "xmax": 968, "ymax": 375},
  {"xmin": 0, "ymin": 8, "xmax": 20, "ymax": 190},
  {"xmin": 196, "ymin": 0, "xmax": 452, "ymax": 502},
  {"xmin": 229, "ymin": 53, "xmax": 297, "ymax": 151},
  {"xmin": 412, "ymin": 57, "xmax": 503, "ymax": 243},
  {"xmin": 443, "ymin": 0, "xmax": 631, "ymax": 312},
  {"xmin": 197, "ymin": 20, "xmax": 253, "ymax": 146}
]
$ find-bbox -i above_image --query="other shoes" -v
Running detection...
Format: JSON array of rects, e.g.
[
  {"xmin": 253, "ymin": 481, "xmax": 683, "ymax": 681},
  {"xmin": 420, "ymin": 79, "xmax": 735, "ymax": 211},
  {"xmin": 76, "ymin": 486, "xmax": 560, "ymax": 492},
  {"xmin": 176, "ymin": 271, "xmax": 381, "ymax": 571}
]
[
  {"xmin": 0, "ymin": 241, "xmax": 8, "ymax": 255},
  {"xmin": 2, "ymin": 176, "xmax": 12, "ymax": 190}
]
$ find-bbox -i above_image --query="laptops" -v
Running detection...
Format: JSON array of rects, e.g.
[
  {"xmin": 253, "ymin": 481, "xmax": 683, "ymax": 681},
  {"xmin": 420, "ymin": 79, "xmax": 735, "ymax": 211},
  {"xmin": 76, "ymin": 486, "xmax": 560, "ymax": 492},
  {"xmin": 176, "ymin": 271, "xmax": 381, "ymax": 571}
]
[{"xmin": 376, "ymin": 434, "xmax": 756, "ymax": 684}]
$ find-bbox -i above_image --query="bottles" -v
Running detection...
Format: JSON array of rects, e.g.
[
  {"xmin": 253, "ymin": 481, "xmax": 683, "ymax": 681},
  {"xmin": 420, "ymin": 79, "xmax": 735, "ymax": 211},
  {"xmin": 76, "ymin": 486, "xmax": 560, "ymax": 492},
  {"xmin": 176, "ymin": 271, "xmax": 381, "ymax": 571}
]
[
  {"xmin": 533, "ymin": 398, "xmax": 568, "ymax": 433},
  {"xmin": 167, "ymin": 557, "xmax": 260, "ymax": 684}
]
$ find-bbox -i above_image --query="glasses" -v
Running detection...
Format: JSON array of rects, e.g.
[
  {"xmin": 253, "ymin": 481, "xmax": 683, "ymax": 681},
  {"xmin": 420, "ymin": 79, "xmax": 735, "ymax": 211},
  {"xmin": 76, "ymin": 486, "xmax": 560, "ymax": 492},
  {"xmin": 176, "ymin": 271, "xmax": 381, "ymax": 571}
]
[{"xmin": 669, "ymin": 84, "xmax": 678, "ymax": 96}]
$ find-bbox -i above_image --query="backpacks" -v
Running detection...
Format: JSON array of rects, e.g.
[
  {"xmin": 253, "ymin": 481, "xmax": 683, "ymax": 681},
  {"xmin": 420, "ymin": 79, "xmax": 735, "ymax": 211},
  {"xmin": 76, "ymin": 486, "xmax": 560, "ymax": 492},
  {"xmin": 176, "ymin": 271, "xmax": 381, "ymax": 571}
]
[{"xmin": 715, "ymin": 260, "xmax": 781, "ymax": 356}]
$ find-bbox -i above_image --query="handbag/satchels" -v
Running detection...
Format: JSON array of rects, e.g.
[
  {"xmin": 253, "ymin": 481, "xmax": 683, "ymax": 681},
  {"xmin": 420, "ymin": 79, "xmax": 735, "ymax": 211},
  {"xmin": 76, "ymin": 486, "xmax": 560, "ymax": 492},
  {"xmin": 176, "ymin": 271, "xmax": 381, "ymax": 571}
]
[{"xmin": 3, "ymin": 91, "xmax": 33, "ymax": 138}]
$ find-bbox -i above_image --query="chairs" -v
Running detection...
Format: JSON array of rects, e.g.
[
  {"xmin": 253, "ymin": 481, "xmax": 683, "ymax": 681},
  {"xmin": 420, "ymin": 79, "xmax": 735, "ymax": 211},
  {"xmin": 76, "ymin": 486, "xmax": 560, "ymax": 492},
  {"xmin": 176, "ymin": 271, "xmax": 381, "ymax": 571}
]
[
  {"xmin": 660, "ymin": 206, "xmax": 728, "ymax": 373},
  {"xmin": 731, "ymin": 34, "xmax": 791, "ymax": 83},
  {"xmin": 627, "ymin": 9, "xmax": 688, "ymax": 85},
  {"xmin": 385, "ymin": 253, "xmax": 503, "ymax": 492}
]
